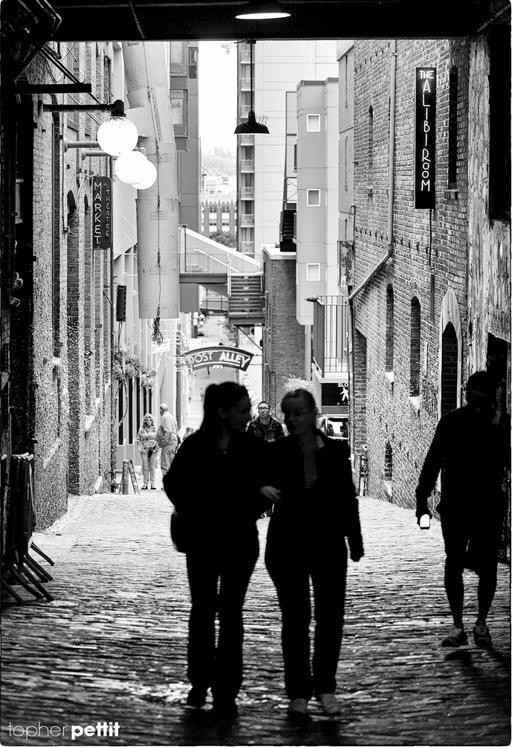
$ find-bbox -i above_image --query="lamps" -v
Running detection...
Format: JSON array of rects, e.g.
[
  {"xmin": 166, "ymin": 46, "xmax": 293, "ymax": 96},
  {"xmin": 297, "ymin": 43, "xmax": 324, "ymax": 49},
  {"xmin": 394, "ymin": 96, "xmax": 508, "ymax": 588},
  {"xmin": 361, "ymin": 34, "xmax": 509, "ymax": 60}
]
[
  {"xmin": 234, "ymin": 38, "xmax": 270, "ymax": 136},
  {"xmin": 44, "ymin": 99, "xmax": 155, "ymax": 197}
]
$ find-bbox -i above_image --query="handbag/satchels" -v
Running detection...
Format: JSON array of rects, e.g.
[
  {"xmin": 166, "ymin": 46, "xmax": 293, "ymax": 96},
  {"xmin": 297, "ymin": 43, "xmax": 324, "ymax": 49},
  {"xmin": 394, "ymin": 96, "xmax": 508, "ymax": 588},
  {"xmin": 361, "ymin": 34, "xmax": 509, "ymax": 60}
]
[{"xmin": 172, "ymin": 513, "xmax": 187, "ymax": 552}]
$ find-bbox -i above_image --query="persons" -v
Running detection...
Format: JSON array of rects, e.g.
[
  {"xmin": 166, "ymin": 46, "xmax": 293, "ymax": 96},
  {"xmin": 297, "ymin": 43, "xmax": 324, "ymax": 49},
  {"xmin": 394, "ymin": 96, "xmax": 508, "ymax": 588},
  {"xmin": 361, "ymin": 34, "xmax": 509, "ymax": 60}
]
[
  {"xmin": 162, "ymin": 380, "xmax": 278, "ymax": 713},
  {"xmin": 261, "ymin": 389, "xmax": 364, "ymax": 718},
  {"xmin": 415, "ymin": 371, "xmax": 509, "ymax": 648},
  {"xmin": 137, "ymin": 401, "xmax": 286, "ymax": 490}
]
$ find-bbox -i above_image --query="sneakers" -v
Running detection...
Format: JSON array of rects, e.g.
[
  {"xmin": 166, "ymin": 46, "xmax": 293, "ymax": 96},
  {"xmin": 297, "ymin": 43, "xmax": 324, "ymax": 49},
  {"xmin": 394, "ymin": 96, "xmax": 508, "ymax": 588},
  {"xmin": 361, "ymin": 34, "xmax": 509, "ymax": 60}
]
[
  {"xmin": 442, "ymin": 627, "xmax": 468, "ymax": 647},
  {"xmin": 312, "ymin": 692, "xmax": 339, "ymax": 713},
  {"xmin": 473, "ymin": 625, "xmax": 491, "ymax": 642},
  {"xmin": 289, "ymin": 699, "xmax": 308, "ymax": 712}
]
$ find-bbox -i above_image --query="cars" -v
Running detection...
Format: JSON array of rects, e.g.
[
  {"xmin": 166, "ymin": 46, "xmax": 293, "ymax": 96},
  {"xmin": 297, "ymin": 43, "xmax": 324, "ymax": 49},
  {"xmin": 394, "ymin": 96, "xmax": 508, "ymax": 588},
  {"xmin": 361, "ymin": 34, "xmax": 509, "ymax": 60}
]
[{"xmin": 318, "ymin": 414, "xmax": 349, "ymax": 445}]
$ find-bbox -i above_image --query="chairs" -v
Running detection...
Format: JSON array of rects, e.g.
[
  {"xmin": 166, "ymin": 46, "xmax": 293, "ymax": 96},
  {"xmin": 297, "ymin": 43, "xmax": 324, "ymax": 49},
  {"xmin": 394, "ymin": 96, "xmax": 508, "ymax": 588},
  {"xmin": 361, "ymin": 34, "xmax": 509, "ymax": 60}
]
[{"xmin": 5, "ymin": 447, "xmax": 59, "ymax": 606}]
